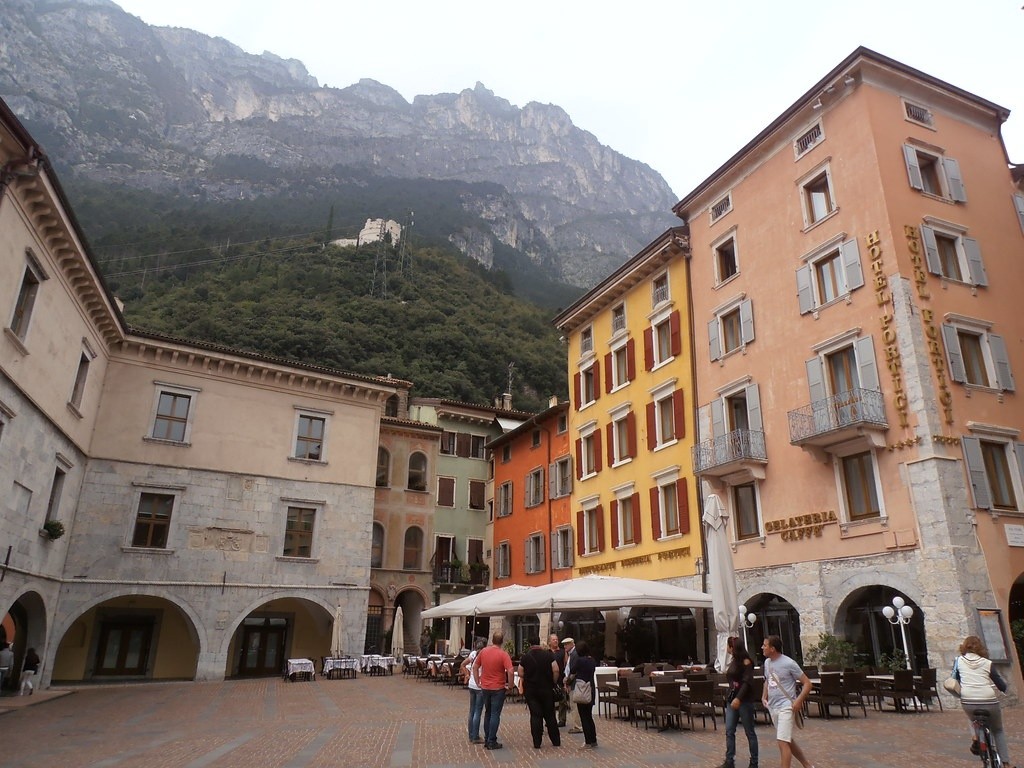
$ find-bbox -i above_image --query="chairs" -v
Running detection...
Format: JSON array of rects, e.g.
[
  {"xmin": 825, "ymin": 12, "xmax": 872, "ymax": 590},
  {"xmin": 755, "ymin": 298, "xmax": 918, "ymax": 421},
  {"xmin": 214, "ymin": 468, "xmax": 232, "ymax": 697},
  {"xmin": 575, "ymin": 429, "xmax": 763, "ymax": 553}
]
[{"xmin": 282, "ymin": 653, "xmax": 943, "ymax": 733}]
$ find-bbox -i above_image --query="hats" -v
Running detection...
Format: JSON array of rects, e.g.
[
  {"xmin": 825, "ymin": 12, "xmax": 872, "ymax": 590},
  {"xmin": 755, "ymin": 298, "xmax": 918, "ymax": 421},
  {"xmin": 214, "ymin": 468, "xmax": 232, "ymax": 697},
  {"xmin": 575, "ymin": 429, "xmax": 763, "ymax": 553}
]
[{"xmin": 560, "ymin": 637, "xmax": 574, "ymax": 643}]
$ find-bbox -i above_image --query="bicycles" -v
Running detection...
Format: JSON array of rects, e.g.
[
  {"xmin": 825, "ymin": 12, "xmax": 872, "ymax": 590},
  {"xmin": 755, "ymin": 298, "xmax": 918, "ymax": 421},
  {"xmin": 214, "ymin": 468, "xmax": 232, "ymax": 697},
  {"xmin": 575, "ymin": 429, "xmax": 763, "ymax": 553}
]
[{"xmin": 970, "ymin": 708, "xmax": 1001, "ymax": 768}]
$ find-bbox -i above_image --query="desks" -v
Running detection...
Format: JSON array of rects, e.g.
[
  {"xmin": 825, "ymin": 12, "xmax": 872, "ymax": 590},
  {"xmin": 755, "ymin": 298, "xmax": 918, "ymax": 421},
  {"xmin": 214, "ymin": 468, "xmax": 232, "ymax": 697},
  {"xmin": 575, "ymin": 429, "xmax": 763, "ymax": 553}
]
[
  {"xmin": 442, "ymin": 663, "xmax": 461, "ymax": 685},
  {"xmin": 865, "ymin": 675, "xmax": 922, "ymax": 713},
  {"xmin": 605, "ymin": 682, "xmax": 651, "ymax": 722},
  {"xmin": 287, "ymin": 658, "xmax": 315, "ymax": 682},
  {"xmin": 638, "ymin": 686, "xmax": 691, "ymax": 732},
  {"xmin": 367, "ymin": 657, "xmax": 398, "ymax": 676},
  {"xmin": 719, "ymin": 682, "xmax": 757, "ymax": 724},
  {"xmin": 428, "ymin": 661, "xmax": 444, "ymax": 681},
  {"xmin": 796, "ymin": 677, "xmax": 844, "ymax": 719},
  {"xmin": 323, "ymin": 660, "xmax": 361, "ymax": 678}
]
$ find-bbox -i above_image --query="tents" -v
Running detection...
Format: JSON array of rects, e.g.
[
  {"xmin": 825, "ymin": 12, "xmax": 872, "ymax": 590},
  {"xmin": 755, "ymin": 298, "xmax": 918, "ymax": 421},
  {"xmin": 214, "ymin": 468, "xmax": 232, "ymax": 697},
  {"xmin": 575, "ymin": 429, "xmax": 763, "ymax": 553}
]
[{"xmin": 420, "ymin": 574, "xmax": 709, "ymax": 651}]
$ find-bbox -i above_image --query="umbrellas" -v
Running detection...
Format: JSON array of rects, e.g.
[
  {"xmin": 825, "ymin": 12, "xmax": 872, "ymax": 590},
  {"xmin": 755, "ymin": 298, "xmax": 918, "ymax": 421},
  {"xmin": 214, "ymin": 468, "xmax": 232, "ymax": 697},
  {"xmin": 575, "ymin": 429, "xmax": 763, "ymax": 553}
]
[
  {"xmin": 702, "ymin": 494, "xmax": 740, "ymax": 675},
  {"xmin": 391, "ymin": 606, "xmax": 403, "ymax": 663}
]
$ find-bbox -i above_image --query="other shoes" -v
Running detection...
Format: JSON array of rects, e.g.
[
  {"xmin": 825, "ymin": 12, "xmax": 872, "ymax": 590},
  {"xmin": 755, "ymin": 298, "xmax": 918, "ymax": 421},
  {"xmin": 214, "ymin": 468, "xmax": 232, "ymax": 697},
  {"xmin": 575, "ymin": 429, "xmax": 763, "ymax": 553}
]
[
  {"xmin": 970, "ymin": 742, "xmax": 980, "ymax": 755},
  {"xmin": 470, "ymin": 737, "xmax": 485, "ymax": 744},
  {"xmin": 556, "ymin": 722, "xmax": 565, "ymax": 726},
  {"xmin": 579, "ymin": 741, "xmax": 598, "ymax": 749},
  {"xmin": 716, "ymin": 761, "xmax": 734, "ymax": 768},
  {"xmin": 484, "ymin": 740, "xmax": 502, "ymax": 750},
  {"xmin": 747, "ymin": 763, "xmax": 758, "ymax": 768},
  {"xmin": 568, "ymin": 727, "xmax": 583, "ymax": 733}
]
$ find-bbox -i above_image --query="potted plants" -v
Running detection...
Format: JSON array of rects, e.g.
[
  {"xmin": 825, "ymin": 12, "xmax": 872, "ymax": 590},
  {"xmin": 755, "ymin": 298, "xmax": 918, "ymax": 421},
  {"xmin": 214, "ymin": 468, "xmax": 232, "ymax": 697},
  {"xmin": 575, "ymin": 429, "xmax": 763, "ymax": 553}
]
[
  {"xmin": 445, "ymin": 560, "xmax": 463, "ymax": 583},
  {"xmin": 819, "ymin": 632, "xmax": 843, "ymax": 672},
  {"xmin": 799, "ymin": 643, "xmax": 821, "ymax": 679},
  {"xmin": 469, "ymin": 563, "xmax": 489, "ymax": 584}
]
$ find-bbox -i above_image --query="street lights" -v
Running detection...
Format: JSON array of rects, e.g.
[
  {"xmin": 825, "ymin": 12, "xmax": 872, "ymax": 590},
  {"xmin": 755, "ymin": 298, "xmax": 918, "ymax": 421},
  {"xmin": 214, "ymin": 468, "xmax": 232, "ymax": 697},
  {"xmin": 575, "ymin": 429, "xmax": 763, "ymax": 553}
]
[
  {"xmin": 883, "ymin": 596, "xmax": 913, "ymax": 669},
  {"xmin": 548, "ymin": 615, "xmax": 563, "ymax": 636},
  {"xmin": 739, "ymin": 605, "xmax": 757, "ymax": 653}
]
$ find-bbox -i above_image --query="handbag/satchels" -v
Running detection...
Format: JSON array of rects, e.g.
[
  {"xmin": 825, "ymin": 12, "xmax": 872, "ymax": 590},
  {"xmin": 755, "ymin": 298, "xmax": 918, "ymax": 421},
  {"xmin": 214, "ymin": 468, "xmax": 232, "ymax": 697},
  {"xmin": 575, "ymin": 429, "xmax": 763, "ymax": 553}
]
[
  {"xmin": 795, "ymin": 709, "xmax": 805, "ymax": 729},
  {"xmin": 944, "ymin": 656, "xmax": 961, "ymax": 697},
  {"xmin": 571, "ymin": 680, "xmax": 592, "ymax": 704},
  {"xmin": 551, "ymin": 684, "xmax": 564, "ymax": 702}
]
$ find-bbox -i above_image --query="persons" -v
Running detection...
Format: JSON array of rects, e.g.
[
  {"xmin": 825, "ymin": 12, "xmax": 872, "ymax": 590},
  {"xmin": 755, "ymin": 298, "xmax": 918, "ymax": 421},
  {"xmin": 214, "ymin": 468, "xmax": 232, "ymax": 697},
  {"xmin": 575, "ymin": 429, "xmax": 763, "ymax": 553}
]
[
  {"xmin": 953, "ymin": 636, "xmax": 1017, "ymax": 768},
  {"xmin": 517, "ymin": 633, "xmax": 561, "ymax": 748},
  {"xmin": 461, "ymin": 638, "xmax": 487, "ymax": 744},
  {"xmin": 547, "ymin": 634, "xmax": 567, "ymax": 727},
  {"xmin": 565, "ymin": 641, "xmax": 599, "ymax": 750},
  {"xmin": 0, "ymin": 642, "xmax": 14, "ymax": 696},
  {"xmin": 420, "ymin": 630, "xmax": 431, "ymax": 657},
  {"xmin": 472, "ymin": 632, "xmax": 514, "ymax": 749},
  {"xmin": 715, "ymin": 637, "xmax": 759, "ymax": 768},
  {"xmin": 561, "ymin": 638, "xmax": 584, "ymax": 734},
  {"xmin": 20, "ymin": 648, "xmax": 40, "ymax": 695},
  {"xmin": 762, "ymin": 635, "xmax": 812, "ymax": 768}
]
